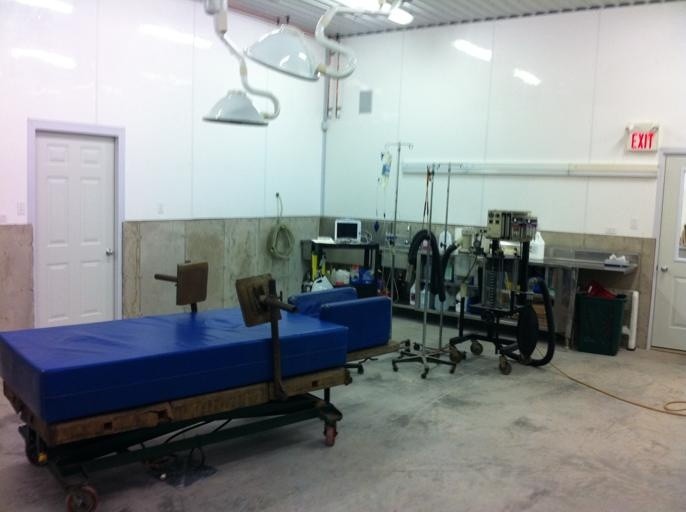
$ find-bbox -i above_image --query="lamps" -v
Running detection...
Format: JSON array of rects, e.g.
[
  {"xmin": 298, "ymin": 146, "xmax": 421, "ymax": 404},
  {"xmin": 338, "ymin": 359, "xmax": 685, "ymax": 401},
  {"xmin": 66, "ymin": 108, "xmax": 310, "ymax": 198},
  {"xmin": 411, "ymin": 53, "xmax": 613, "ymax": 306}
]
[{"xmin": 199, "ymin": 0, "xmax": 399, "ymax": 126}]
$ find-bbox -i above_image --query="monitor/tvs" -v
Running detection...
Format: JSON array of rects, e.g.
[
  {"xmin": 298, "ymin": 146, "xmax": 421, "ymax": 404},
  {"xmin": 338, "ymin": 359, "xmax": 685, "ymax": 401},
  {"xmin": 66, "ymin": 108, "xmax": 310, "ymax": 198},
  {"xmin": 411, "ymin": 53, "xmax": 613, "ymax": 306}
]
[{"xmin": 335, "ymin": 219, "xmax": 361, "ymax": 242}]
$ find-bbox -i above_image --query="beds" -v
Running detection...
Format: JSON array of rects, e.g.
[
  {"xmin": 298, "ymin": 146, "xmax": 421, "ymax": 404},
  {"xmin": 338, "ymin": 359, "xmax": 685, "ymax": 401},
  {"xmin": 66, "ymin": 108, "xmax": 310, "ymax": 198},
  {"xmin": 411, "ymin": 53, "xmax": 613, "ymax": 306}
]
[{"xmin": 1, "ymin": 306, "xmax": 348, "ymax": 443}]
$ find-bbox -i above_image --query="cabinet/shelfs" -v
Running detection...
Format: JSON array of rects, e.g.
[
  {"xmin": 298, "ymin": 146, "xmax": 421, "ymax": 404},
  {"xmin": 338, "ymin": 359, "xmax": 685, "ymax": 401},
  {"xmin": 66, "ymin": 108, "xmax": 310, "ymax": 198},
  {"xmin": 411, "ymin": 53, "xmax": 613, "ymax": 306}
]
[{"xmin": 379, "ymin": 240, "xmax": 639, "ymax": 356}]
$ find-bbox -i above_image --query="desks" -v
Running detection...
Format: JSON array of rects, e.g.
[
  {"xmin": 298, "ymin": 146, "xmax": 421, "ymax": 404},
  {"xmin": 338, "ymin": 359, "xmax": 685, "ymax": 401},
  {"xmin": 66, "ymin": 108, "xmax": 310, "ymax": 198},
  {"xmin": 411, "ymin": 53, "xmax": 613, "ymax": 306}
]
[{"xmin": 309, "ymin": 238, "xmax": 380, "ymax": 291}]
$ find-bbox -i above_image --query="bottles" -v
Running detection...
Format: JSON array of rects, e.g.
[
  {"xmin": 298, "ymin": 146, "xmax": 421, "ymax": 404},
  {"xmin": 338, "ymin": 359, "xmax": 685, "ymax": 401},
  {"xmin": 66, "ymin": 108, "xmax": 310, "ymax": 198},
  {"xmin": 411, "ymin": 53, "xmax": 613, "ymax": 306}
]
[{"xmin": 328, "ymin": 264, "xmax": 359, "ymax": 285}]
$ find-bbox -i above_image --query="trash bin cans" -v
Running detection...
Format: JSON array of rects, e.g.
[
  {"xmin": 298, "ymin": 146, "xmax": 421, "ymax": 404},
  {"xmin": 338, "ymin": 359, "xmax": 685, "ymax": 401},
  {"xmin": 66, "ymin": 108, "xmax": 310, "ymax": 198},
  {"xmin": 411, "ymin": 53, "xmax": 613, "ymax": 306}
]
[{"xmin": 571, "ymin": 291, "xmax": 628, "ymax": 356}]
